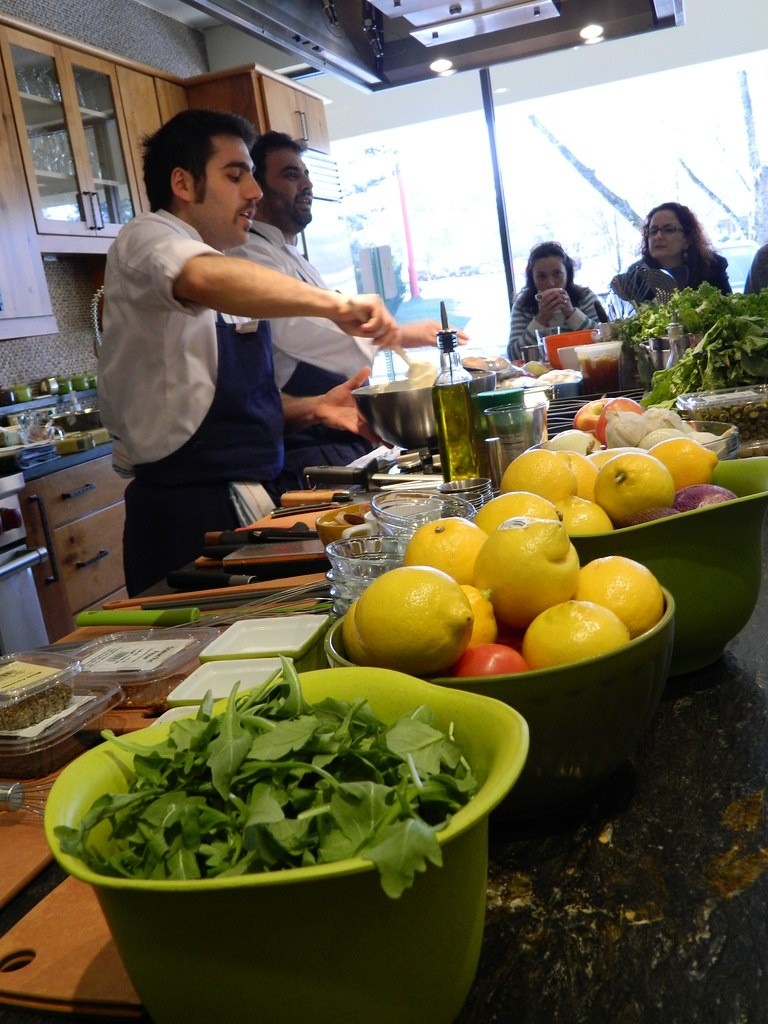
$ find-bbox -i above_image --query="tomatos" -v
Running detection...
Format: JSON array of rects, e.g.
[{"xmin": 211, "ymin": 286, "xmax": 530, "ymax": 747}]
[{"xmin": 444, "ymin": 643, "xmax": 530, "ymax": 677}]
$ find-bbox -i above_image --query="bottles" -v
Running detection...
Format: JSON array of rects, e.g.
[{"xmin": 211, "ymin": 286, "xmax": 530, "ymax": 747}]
[
  {"xmin": 432, "ymin": 300, "xmax": 482, "ymax": 487},
  {"xmin": 664, "ymin": 309, "xmax": 690, "ymax": 369}
]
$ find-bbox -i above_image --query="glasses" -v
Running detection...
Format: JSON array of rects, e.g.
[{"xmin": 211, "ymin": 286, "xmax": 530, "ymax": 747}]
[{"xmin": 645, "ymin": 225, "xmax": 683, "ymax": 236}]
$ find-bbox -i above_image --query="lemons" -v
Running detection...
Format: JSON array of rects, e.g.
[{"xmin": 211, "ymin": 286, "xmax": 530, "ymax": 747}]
[
  {"xmin": 473, "ymin": 438, "xmax": 720, "ymax": 536},
  {"xmin": 341, "ymin": 515, "xmax": 663, "ymax": 677}
]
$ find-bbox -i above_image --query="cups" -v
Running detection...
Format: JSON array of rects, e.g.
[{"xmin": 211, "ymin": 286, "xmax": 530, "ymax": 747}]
[
  {"xmin": 535, "ymin": 326, "xmax": 561, "ymax": 363},
  {"xmin": 487, "ymin": 405, "xmax": 549, "ymax": 490},
  {"xmin": 0, "ymin": 371, "xmax": 98, "ymax": 405},
  {"xmin": 536, "ymin": 289, "xmax": 563, "ymax": 312}
]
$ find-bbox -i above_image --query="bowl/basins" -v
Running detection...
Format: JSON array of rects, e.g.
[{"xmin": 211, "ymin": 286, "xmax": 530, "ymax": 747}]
[
  {"xmin": 674, "ymin": 380, "xmax": 768, "ymax": 452},
  {"xmin": 519, "ymin": 419, "xmax": 742, "ymax": 460},
  {"xmin": 52, "ymin": 407, "xmax": 104, "ymax": 435},
  {"xmin": 639, "ymin": 334, "xmax": 703, "ymax": 372},
  {"xmin": 323, "ymin": 473, "xmax": 490, "ymax": 619},
  {"xmin": 0, "ymin": 627, "xmax": 223, "ymax": 781},
  {"xmin": 324, "ymin": 563, "xmax": 674, "ymax": 817},
  {"xmin": 542, "ymin": 330, "xmax": 599, "ymax": 399},
  {"xmin": 507, "ymin": 447, "xmax": 768, "ymax": 683},
  {"xmin": 45, "ymin": 666, "xmax": 532, "ymax": 1023}
]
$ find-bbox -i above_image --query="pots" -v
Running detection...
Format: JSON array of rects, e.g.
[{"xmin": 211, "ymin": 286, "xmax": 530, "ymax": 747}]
[{"xmin": 350, "ymin": 369, "xmax": 499, "ymax": 448}]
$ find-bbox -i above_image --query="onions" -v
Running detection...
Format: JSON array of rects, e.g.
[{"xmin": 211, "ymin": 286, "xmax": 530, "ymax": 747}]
[{"xmin": 544, "ymin": 427, "xmax": 684, "ymax": 452}]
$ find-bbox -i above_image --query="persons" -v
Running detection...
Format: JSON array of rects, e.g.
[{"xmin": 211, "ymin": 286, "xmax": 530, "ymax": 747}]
[
  {"xmin": 607, "ymin": 202, "xmax": 731, "ymax": 321},
  {"xmin": 227, "ymin": 129, "xmax": 468, "ymax": 497},
  {"xmin": 506, "ymin": 241, "xmax": 610, "ymax": 362},
  {"xmin": 744, "ymin": 243, "xmax": 768, "ymax": 296},
  {"xmin": 96, "ymin": 109, "xmax": 400, "ymax": 600}
]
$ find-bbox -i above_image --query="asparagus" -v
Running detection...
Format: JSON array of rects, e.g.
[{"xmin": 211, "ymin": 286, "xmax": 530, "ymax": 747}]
[{"xmin": 688, "ymin": 399, "xmax": 768, "ymax": 445}]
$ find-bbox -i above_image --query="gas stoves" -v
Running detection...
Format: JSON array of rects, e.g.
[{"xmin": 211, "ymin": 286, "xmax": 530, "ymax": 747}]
[{"xmin": 312, "ymin": 442, "xmax": 441, "ymax": 494}]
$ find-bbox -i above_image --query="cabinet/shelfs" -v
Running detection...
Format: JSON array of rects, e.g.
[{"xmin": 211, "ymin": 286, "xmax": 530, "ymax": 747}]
[
  {"xmin": 23, "ymin": 455, "xmax": 135, "ymax": 645},
  {"xmin": 0, "ymin": 16, "xmax": 349, "ymax": 344}
]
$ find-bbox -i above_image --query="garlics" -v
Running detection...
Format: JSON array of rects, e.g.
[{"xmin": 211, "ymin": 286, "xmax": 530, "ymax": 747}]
[{"xmin": 604, "ymin": 408, "xmax": 740, "ymax": 459}]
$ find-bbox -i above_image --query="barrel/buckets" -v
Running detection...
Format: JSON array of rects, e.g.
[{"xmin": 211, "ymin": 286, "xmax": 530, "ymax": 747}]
[{"xmin": 576, "ymin": 341, "xmax": 623, "ymax": 392}]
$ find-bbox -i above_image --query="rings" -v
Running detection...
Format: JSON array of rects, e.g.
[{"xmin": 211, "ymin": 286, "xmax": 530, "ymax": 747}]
[{"xmin": 564, "ymin": 300, "xmax": 567, "ymax": 304}]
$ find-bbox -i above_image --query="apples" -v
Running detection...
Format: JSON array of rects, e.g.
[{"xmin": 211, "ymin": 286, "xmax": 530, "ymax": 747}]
[{"xmin": 573, "ymin": 397, "xmax": 646, "ymax": 448}]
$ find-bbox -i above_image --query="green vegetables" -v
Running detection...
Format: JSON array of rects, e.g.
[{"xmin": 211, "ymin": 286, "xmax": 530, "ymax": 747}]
[
  {"xmin": 50, "ymin": 651, "xmax": 478, "ymax": 899},
  {"xmin": 612, "ymin": 283, "xmax": 768, "ymax": 411}
]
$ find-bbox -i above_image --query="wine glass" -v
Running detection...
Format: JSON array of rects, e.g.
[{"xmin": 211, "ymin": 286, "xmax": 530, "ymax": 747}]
[{"xmin": 14, "ymin": 62, "xmax": 126, "ymax": 182}]
